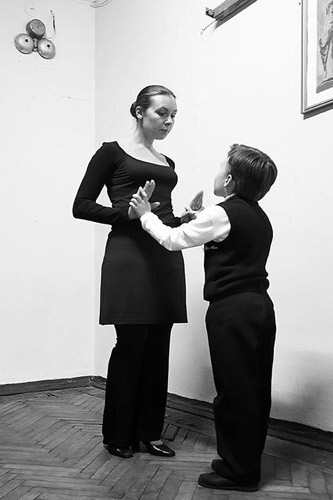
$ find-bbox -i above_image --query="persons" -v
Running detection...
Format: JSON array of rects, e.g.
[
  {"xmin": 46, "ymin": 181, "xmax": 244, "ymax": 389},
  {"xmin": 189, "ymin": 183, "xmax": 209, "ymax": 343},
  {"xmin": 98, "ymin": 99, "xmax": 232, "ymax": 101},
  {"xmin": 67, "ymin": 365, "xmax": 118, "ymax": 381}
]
[
  {"xmin": 72, "ymin": 85, "xmax": 203, "ymax": 458},
  {"xmin": 128, "ymin": 144, "xmax": 277, "ymax": 492}
]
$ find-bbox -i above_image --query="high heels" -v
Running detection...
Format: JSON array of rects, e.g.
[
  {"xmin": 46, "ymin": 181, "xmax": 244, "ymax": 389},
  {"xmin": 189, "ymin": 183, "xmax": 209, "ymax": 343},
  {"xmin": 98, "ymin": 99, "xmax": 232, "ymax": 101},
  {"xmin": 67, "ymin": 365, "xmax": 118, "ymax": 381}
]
[
  {"xmin": 103, "ymin": 435, "xmax": 134, "ymax": 458},
  {"xmin": 131, "ymin": 433, "xmax": 175, "ymax": 457}
]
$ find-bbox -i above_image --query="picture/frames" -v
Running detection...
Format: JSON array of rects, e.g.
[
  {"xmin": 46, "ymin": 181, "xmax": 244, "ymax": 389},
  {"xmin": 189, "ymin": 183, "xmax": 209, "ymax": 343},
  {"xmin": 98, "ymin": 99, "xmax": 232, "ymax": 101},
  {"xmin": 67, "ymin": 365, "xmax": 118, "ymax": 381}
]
[{"xmin": 300, "ymin": 0, "xmax": 333, "ymax": 115}]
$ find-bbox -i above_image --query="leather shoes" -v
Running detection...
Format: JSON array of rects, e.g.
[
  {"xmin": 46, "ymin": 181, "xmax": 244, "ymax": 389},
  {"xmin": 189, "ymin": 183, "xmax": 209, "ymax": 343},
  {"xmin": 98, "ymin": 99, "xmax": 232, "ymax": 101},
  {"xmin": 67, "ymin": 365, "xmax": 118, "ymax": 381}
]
[
  {"xmin": 211, "ymin": 459, "xmax": 233, "ymax": 471},
  {"xmin": 198, "ymin": 471, "xmax": 261, "ymax": 492}
]
